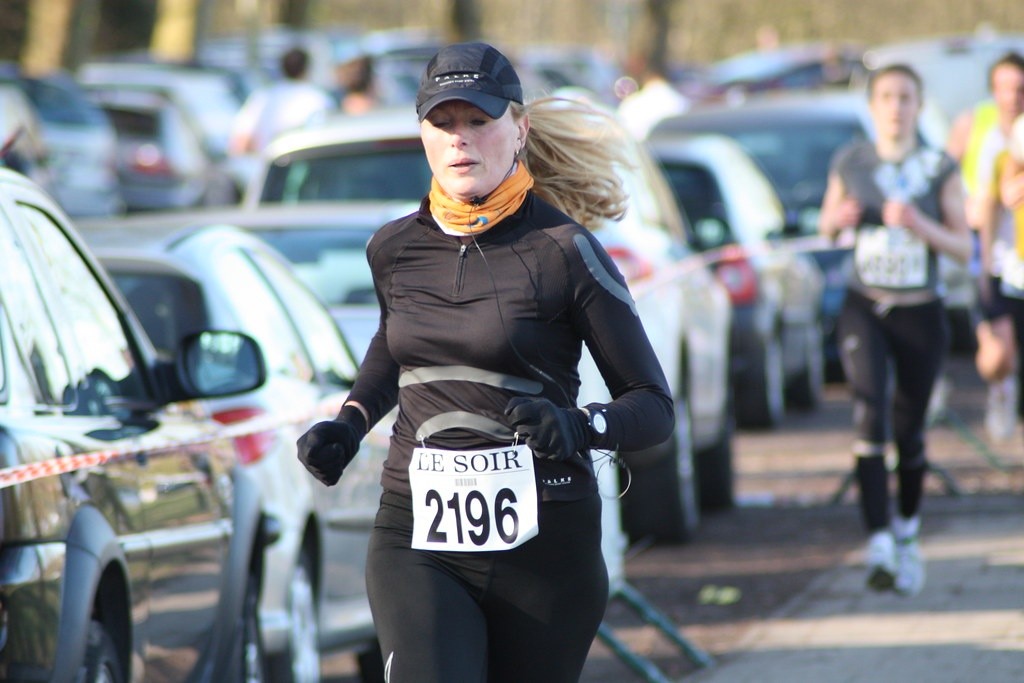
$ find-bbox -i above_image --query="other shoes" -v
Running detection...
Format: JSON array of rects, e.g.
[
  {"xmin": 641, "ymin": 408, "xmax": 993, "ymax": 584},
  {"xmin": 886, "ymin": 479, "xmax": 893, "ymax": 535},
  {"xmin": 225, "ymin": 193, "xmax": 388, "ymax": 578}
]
[
  {"xmin": 987, "ymin": 379, "xmax": 1017, "ymax": 442},
  {"xmin": 864, "ymin": 550, "xmax": 895, "ymax": 591},
  {"xmin": 897, "ymin": 540, "xmax": 926, "ymax": 597}
]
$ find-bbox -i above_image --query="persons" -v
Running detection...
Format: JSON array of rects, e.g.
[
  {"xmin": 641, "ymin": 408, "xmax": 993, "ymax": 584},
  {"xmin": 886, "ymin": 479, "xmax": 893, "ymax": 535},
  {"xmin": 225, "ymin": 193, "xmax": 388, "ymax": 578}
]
[
  {"xmin": 819, "ymin": 39, "xmax": 1024, "ymax": 597},
  {"xmin": 296, "ymin": 42, "xmax": 676, "ymax": 683},
  {"xmin": 231, "ymin": 43, "xmax": 391, "ymax": 203}
]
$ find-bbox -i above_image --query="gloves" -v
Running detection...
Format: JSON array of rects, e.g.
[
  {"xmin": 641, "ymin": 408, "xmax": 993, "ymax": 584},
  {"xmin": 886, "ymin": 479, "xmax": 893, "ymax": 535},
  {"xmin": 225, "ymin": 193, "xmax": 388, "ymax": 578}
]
[
  {"xmin": 297, "ymin": 421, "xmax": 358, "ymax": 487},
  {"xmin": 504, "ymin": 396, "xmax": 591, "ymax": 460}
]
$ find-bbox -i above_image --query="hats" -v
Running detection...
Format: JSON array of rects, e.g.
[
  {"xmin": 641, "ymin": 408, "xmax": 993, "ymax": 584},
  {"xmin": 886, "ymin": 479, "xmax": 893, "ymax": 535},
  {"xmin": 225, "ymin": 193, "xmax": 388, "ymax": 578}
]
[{"xmin": 414, "ymin": 43, "xmax": 524, "ymax": 123}]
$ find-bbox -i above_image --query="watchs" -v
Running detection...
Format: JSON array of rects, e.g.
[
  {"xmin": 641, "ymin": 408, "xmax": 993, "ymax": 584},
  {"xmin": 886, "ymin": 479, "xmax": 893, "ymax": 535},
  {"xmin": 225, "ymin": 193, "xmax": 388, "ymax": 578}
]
[{"xmin": 582, "ymin": 405, "xmax": 608, "ymax": 450}]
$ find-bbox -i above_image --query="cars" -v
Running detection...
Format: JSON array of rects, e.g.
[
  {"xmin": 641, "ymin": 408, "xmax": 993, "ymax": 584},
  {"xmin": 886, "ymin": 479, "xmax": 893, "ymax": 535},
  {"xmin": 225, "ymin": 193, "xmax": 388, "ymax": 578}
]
[{"xmin": 0, "ymin": 27, "xmax": 1023, "ymax": 683}]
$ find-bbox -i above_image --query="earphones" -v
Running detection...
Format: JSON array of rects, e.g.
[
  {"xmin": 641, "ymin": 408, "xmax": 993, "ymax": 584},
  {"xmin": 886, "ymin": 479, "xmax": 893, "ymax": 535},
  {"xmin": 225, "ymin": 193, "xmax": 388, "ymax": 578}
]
[{"xmin": 517, "ymin": 137, "xmax": 522, "ymax": 144}]
